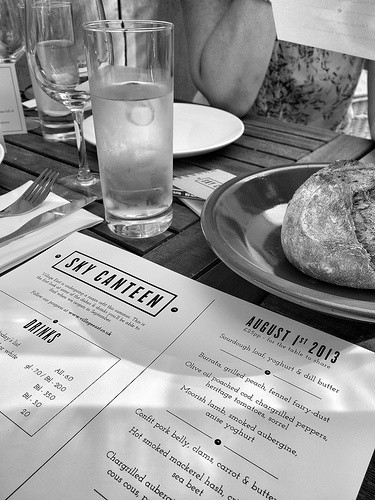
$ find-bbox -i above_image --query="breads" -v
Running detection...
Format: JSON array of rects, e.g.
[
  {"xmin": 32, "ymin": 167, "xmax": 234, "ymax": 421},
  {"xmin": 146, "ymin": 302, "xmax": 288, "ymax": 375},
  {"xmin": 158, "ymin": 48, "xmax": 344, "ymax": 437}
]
[{"xmin": 281, "ymin": 159, "xmax": 375, "ymax": 289}]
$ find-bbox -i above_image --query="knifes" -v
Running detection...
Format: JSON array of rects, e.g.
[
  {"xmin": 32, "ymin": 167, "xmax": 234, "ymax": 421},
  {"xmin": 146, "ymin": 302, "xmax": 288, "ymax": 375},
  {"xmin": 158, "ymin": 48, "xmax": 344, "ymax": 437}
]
[{"xmin": 1, "ymin": 195, "xmax": 99, "ymax": 252}]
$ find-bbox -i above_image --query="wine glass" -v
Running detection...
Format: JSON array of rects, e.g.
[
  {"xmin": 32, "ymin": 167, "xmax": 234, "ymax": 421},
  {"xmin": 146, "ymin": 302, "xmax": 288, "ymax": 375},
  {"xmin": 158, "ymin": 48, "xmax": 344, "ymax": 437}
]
[{"xmin": 23, "ymin": 0, "xmax": 115, "ymax": 202}]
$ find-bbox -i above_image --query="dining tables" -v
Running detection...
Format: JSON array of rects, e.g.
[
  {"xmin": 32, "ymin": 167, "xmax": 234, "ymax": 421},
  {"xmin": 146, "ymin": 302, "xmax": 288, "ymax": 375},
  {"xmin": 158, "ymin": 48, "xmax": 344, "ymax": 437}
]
[{"xmin": 0, "ymin": 88, "xmax": 375, "ymax": 500}]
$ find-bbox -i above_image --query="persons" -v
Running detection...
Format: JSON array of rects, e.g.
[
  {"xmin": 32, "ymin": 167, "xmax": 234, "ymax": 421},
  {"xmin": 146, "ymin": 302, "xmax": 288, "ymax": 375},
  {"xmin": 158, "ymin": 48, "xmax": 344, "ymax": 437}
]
[{"xmin": 66, "ymin": 0, "xmax": 375, "ymax": 142}]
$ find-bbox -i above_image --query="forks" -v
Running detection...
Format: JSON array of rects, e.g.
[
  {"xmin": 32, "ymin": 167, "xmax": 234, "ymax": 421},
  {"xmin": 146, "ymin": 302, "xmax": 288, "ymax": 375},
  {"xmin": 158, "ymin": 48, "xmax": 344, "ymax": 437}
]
[{"xmin": 1, "ymin": 165, "xmax": 61, "ymax": 217}]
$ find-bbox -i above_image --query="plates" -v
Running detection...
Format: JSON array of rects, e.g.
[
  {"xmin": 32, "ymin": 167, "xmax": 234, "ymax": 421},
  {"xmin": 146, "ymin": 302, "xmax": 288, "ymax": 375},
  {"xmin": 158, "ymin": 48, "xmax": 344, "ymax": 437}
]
[
  {"xmin": 200, "ymin": 161, "xmax": 375, "ymax": 324},
  {"xmin": 76, "ymin": 101, "xmax": 248, "ymax": 158}
]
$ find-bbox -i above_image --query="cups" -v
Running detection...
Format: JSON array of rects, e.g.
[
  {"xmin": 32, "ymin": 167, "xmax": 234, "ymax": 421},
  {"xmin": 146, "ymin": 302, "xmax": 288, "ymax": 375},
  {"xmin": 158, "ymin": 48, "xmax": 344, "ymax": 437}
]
[{"xmin": 81, "ymin": 20, "xmax": 176, "ymax": 242}]
